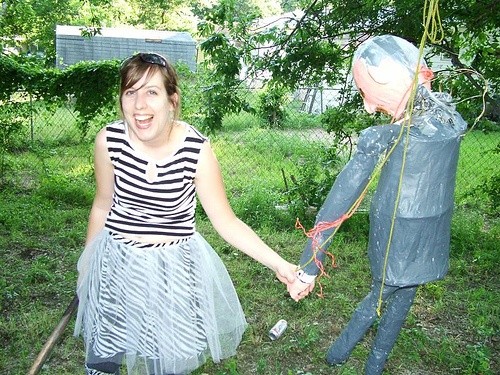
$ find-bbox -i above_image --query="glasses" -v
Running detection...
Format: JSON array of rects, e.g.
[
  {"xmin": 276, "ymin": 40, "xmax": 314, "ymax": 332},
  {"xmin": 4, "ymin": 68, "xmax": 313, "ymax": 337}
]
[{"xmin": 120, "ymin": 52, "xmax": 170, "ymax": 74}]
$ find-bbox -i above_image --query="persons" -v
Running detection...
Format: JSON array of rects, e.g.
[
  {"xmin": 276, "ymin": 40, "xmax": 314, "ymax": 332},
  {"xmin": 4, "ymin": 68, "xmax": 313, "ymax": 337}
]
[
  {"xmin": 75, "ymin": 52, "xmax": 315, "ymax": 375},
  {"xmin": 282, "ymin": 34, "xmax": 467, "ymax": 375}
]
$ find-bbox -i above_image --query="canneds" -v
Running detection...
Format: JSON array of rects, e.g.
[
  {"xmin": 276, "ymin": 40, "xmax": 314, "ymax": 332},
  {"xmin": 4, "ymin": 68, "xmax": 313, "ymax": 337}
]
[{"xmin": 269, "ymin": 319, "xmax": 287, "ymax": 340}]
